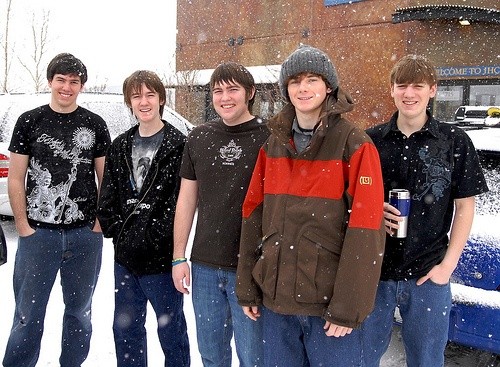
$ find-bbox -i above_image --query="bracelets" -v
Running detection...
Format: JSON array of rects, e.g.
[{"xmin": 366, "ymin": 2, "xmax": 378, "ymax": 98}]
[{"xmin": 172, "ymin": 257, "xmax": 187, "ymax": 265}]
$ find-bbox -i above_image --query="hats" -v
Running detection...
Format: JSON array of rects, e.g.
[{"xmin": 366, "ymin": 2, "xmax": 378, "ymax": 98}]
[{"xmin": 280, "ymin": 46, "xmax": 338, "ymax": 102}]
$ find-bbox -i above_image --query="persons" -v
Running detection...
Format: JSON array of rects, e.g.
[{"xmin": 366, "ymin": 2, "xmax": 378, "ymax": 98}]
[
  {"xmin": 359, "ymin": 54, "xmax": 489, "ymax": 367},
  {"xmin": 95, "ymin": 70, "xmax": 192, "ymax": 367},
  {"xmin": 2, "ymin": 52, "xmax": 112, "ymax": 367},
  {"xmin": 235, "ymin": 44, "xmax": 385, "ymax": 367},
  {"xmin": 171, "ymin": 61, "xmax": 275, "ymax": 367}
]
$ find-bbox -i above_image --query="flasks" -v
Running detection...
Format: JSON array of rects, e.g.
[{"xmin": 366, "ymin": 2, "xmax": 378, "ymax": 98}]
[{"xmin": 389, "ymin": 189, "xmax": 410, "ymax": 238}]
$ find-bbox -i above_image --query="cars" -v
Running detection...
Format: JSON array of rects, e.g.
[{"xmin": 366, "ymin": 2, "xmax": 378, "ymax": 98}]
[
  {"xmin": 394, "ymin": 105, "xmax": 500, "ymax": 356},
  {"xmin": 0, "ymin": 91, "xmax": 195, "ymax": 221}
]
